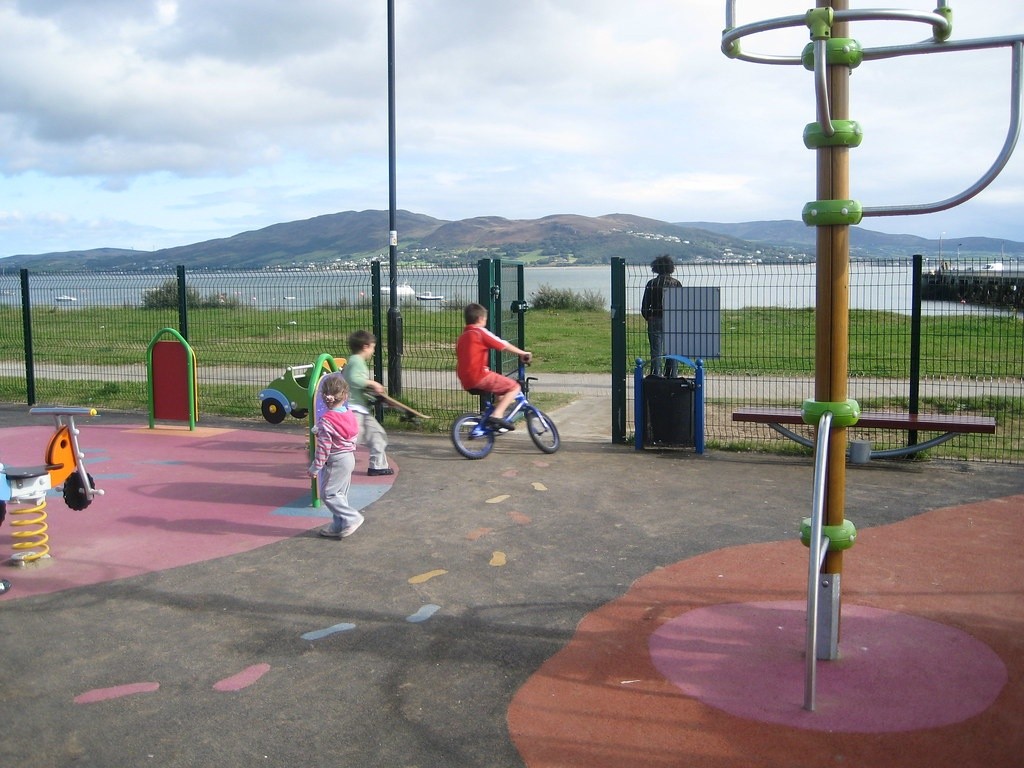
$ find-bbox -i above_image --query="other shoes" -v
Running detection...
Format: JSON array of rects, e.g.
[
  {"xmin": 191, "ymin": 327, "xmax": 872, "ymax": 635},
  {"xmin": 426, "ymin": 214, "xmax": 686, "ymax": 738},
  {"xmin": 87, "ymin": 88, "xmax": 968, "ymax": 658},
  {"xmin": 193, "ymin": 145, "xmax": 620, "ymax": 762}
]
[
  {"xmin": 367, "ymin": 468, "xmax": 394, "ymax": 476},
  {"xmin": 320, "ymin": 529, "xmax": 340, "ymax": 536},
  {"xmin": 489, "ymin": 417, "xmax": 515, "ymax": 430},
  {"xmin": 340, "ymin": 517, "xmax": 364, "ymax": 537}
]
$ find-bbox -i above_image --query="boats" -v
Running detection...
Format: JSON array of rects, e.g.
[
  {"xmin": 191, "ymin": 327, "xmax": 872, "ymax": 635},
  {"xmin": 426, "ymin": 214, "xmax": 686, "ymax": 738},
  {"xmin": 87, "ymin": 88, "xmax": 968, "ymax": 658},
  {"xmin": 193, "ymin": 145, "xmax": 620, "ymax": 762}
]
[
  {"xmin": 55, "ymin": 294, "xmax": 75, "ymax": 301},
  {"xmin": 416, "ymin": 291, "xmax": 444, "ymax": 301},
  {"xmin": 379, "ymin": 285, "xmax": 415, "ymax": 295}
]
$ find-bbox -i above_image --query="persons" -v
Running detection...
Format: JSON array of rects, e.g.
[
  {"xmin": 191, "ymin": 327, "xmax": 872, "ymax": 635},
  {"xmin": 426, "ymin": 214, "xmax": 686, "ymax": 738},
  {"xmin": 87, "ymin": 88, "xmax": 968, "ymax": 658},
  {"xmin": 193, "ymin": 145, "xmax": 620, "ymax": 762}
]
[
  {"xmin": 639, "ymin": 253, "xmax": 683, "ymax": 378},
  {"xmin": 454, "ymin": 303, "xmax": 532, "ymax": 431},
  {"xmin": 342, "ymin": 328, "xmax": 395, "ymax": 475},
  {"xmin": 307, "ymin": 376, "xmax": 364, "ymax": 539}
]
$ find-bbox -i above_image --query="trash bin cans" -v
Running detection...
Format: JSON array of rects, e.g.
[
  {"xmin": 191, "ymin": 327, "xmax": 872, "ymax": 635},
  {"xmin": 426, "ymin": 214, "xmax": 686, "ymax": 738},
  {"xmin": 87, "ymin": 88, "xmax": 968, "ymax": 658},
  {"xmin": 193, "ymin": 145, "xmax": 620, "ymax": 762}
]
[{"xmin": 646, "ymin": 373, "xmax": 696, "ymax": 442}]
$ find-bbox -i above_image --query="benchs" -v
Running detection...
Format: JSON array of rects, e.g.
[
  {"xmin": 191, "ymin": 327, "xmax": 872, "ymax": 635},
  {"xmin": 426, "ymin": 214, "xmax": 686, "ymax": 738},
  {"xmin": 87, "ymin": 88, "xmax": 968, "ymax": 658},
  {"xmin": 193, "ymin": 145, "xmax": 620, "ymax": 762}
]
[{"xmin": 734, "ymin": 407, "xmax": 996, "ymax": 464}]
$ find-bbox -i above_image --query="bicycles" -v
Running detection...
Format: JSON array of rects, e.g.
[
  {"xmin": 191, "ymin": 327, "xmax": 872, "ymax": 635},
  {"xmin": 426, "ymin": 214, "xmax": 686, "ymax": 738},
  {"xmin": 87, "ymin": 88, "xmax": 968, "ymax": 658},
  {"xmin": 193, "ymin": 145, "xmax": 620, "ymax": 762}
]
[{"xmin": 452, "ymin": 354, "xmax": 560, "ymax": 460}]
